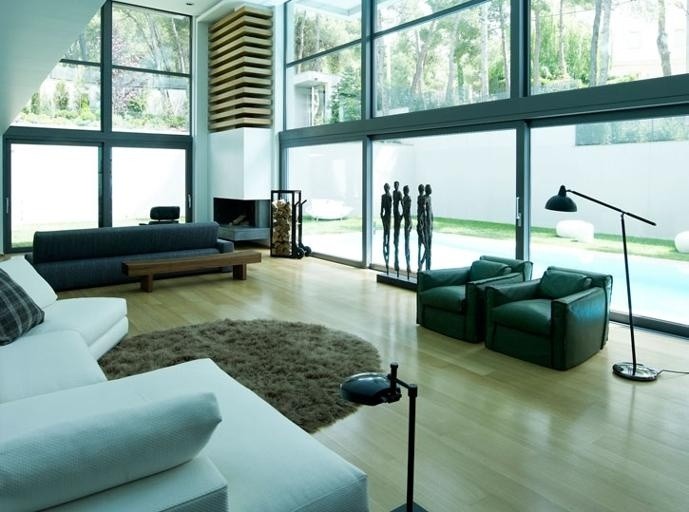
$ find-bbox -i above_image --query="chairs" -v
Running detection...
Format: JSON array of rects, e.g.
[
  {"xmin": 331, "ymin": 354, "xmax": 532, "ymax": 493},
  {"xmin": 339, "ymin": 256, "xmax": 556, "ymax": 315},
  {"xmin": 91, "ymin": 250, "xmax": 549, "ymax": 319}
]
[{"xmin": 139, "ymin": 206, "xmax": 180, "ymax": 225}]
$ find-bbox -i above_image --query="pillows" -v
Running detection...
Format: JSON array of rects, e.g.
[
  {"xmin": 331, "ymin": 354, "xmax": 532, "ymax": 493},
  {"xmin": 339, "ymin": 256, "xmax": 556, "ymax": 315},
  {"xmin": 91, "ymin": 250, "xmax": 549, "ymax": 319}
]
[{"xmin": 0, "ymin": 267, "xmax": 45, "ymax": 346}]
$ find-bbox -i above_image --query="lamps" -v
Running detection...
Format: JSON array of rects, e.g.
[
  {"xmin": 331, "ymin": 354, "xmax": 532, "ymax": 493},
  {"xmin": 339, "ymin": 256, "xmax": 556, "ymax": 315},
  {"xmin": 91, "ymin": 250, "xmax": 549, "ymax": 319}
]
[
  {"xmin": 340, "ymin": 362, "xmax": 430, "ymax": 512},
  {"xmin": 544, "ymin": 184, "xmax": 658, "ymax": 382}
]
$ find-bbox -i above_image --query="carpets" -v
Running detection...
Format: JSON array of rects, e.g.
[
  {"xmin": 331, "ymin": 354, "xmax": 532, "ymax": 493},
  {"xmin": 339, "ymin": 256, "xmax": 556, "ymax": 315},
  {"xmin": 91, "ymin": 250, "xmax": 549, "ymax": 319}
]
[{"xmin": 97, "ymin": 317, "xmax": 386, "ymax": 434}]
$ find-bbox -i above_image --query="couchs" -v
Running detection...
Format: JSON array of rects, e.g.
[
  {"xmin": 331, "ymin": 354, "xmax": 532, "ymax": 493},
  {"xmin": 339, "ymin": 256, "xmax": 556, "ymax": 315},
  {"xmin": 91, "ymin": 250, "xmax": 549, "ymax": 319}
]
[
  {"xmin": 416, "ymin": 255, "xmax": 613, "ymax": 371},
  {"xmin": 24, "ymin": 221, "xmax": 234, "ymax": 292},
  {"xmin": 0, "ymin": 254, "xmax": 371, "ymax": 511}
]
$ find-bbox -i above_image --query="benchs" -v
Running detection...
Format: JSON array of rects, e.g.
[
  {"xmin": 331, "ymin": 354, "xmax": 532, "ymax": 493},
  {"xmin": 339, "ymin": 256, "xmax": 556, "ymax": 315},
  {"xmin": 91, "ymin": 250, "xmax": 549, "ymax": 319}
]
[{"xmin": 121, "ymin": 250, "xmax": 261, "ymax": 293}]
